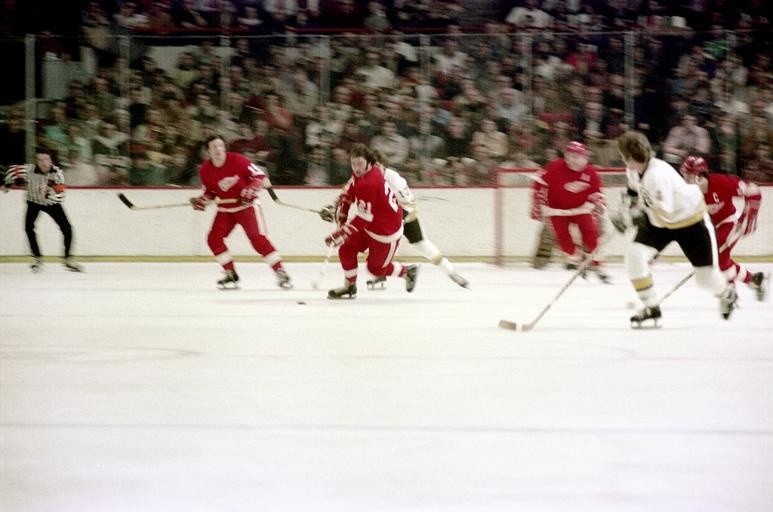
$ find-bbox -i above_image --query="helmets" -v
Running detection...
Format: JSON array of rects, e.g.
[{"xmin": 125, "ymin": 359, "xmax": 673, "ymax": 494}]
[
  {"xmin": 680, "ymin": 156, "xmax": 708, "ymax": 176},
  {"xmin": 564, "ymin": 141, "xmax": 590, "ymax": 154}
]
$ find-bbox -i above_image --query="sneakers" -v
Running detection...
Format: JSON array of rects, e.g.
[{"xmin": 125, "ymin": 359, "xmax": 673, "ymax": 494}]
[
  {"xmin": 274, "ymin": 269, "xmax": 289, "ymax": 287},
  {"xmin": 631, "ymin": 305, "xmax": 661, "ymax": 321},
  {"xmin": 329, "ymin": 264, "xmax": 417, "ymax": 297},
  {"xmin": 218, "ymin": 269, "xmax": 239, "ymax": 284},
  {"xmin": 719, "ymin": 273, "xmax": 764, "ymax": 319},
  {"xmin": 450, "ymin": 271, "xmax": 469, "ymax": 286}
]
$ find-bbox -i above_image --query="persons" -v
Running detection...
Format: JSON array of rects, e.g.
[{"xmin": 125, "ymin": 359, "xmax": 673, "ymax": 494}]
[
  {"xmin": 317, "ymin": 148, "xmax": 472, "ymax": 294},
  {"xmin": 322, "ymin": 141, "xmax": 418, "ymax": 297},
  {"xmin": 0, "ymin": 0, "xmax": 773, "ymax": 193},
  {"xmin": 0, "ymin": 148, "xmax": 85, "ymax": 274},
  {"xmin": 527, "ymin": 139, "xmax": 610, "ymax": 283},
  {"xmin": 187, "ymin": 133, "xmax": 290, "ymax": 287},
  {"xmin": 675, "ymin": 153, "xmax": 766, "ymax": 304},
  {"xmin": 607, "ymin": 130, "xmax": 739, "ymax": 322}
]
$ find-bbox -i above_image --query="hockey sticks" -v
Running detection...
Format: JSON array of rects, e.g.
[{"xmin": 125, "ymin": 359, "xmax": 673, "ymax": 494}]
[
  {"xmin": 119, "ymin": 193, "xmax": 240, "ymax": 211},
  {"xmin": 263, "ymin": 177, "xmax": 323, "ymax": 213},
  {"xmin": 497, "ymin": 224, "xmax": 617, "ymax": 333}
]
